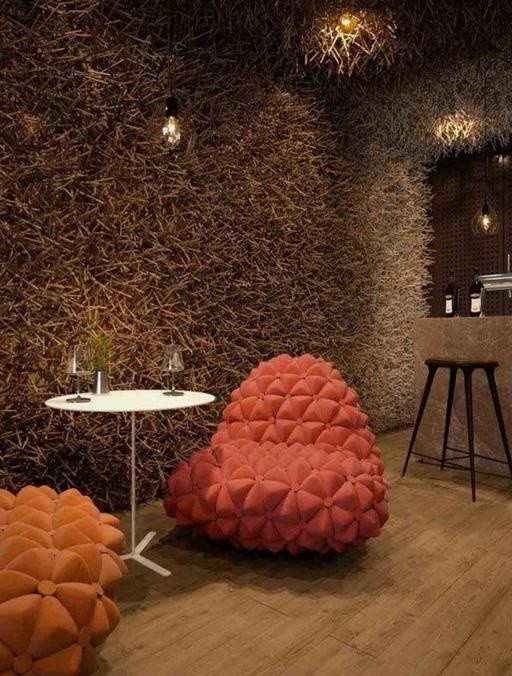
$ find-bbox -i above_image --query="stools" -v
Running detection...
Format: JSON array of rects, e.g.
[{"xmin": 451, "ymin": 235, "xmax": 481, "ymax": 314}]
[
  {"xmin": 0, "ymin": 485, "xmax": 130, "ymax": 676},
  {"xmin": 401, "ymin": 356, "xmax": 512, "ymax": 503}
]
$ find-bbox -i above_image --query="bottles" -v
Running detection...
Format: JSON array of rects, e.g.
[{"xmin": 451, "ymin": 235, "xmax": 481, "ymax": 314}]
[
  {"xmin": 468, "ymin": 268, "xmax": 486, "ymax": 317},
  {"xmin": 444, "ymin": 270, "xmax": 461, "ymax": 317}
]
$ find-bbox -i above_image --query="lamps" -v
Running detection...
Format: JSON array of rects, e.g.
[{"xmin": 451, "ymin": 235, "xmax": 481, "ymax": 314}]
[
  {"xmin": 161, "ymin": 1, "xmax": 183, "ymax": 148},
  {"xmin": 470, "ymin": 1, "xmax": 504, "ymax": 239}
]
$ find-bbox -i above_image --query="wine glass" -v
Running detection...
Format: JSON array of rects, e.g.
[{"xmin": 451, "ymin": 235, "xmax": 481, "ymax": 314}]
[
  {"xmin": 65, "ymin": 343, "xmax": 95, "ymax": 405},
  {"xmin": 161, "ymin": 344, "xmax": 185, "ymax": 396}
]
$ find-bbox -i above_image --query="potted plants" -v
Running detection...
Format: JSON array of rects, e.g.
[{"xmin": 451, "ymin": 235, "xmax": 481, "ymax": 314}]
[{"xmin": 85, "ymin": 334, "xmax": 117, "ymax": 395}]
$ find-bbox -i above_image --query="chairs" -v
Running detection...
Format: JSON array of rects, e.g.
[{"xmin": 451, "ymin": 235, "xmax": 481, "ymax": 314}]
[{"xmin": 162, "ymin": 352, "xmax": 390, "ymax": 556}]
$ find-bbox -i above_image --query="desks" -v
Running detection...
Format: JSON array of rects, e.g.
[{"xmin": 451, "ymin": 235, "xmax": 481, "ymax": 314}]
[{"xmin": 44, "ymin": 389, "xmax": 215, "ymax": 577}]
